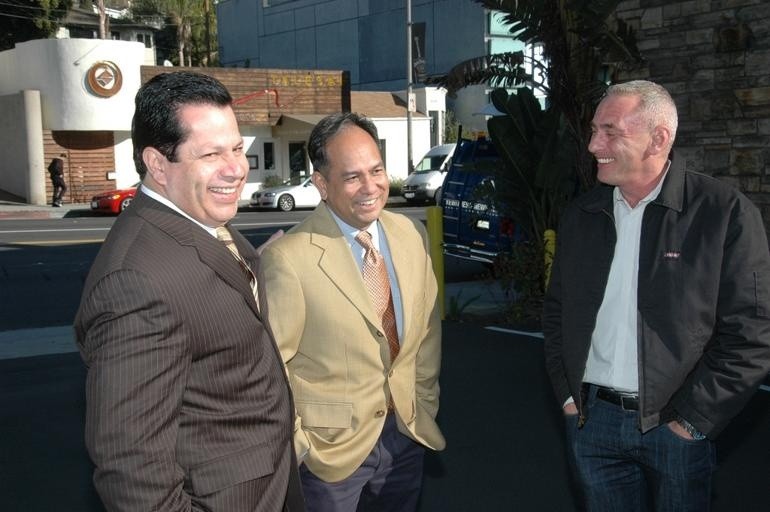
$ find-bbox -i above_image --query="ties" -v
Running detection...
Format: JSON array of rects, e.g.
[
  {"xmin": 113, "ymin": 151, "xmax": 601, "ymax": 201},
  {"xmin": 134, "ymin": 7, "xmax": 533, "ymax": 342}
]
[
  {"xmin": 356, "ymin": 231, "xmax": 400, "ymax": 412},
  {"xmin": 217, "ymin": 224, "xmax": 261, "ymax": 312}
]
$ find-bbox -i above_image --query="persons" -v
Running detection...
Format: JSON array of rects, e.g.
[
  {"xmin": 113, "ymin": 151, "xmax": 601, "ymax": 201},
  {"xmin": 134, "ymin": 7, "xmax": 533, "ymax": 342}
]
[
  {"xmin": 539, "ymin": 76, "xmax": 769, "ymax": 511},
  {"xmin": 47, "ymin": 152, "xmax": 68, "ymax": 208},
  {"xmin": 70, "ymin": 70, "xmax": 309, "ymax": 511},
  {"xmin": 254, "ymin": 109, "xmax": 449, "ymax": 511}
]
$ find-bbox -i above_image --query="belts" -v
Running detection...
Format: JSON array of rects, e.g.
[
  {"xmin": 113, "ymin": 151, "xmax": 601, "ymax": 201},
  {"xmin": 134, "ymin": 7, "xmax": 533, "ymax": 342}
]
[{"xmin": 596, "ymin": 387, "xmax": 640, "ymax": 412}]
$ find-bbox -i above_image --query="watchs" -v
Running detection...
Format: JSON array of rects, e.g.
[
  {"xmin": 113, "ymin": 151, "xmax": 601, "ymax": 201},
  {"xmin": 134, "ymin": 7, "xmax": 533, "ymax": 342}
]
[{"xmin": 676, "ymin": 413, "xmax": 707, "ymax": 441}]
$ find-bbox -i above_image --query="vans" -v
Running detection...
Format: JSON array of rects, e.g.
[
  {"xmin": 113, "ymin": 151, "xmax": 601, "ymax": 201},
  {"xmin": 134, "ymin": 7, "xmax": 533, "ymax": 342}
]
[
  {"xmin": 436, "ymin": 123, "xmax": 528, "ymax": 274},
  {"xmin": 402, "ymin": 142, "xmax": 459, "ymax": 204}
]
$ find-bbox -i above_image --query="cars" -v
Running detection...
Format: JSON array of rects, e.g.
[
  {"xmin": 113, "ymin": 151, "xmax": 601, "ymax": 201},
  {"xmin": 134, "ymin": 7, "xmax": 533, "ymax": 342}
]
[
  {"xmin": 250, "ymin": 175, "xmax": 322, "ymax": 211},
  {"xmin": 91, "ymin": 181, "xmax": 143, "ymax": 214}
]
